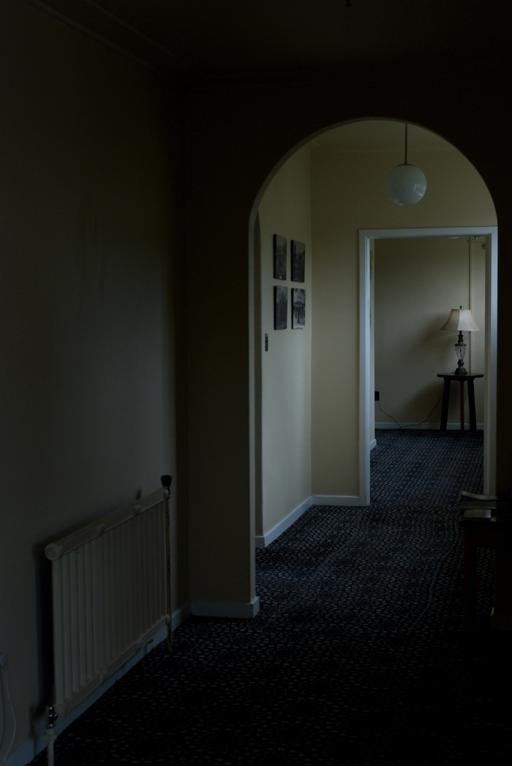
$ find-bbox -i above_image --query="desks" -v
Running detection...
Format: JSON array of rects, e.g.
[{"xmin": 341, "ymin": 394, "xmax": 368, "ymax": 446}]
[{"xmin": 436, "ymin": 372, "xmax": 485, "ymax": 436}]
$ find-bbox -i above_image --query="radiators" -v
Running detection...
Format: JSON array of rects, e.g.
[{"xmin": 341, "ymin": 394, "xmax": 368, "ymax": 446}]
[{"xmin": 31, "ymin": 472, "xmax": 183, "ymax": 766}]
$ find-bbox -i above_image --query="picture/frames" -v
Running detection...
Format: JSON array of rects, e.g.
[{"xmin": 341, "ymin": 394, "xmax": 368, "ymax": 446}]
[
  {"xmin": 274, "ymin": 286, "xmax": 288, "ymax": 330},
  {"xmin": 291, "ymin": 288, "xmax": 306, "ymax": 329},
  {"xmin": 290, "ymin": 239, "xmax": 307, "ymax": 282},
  {"xmin": 274, "ymin": 234, "xmax": 288, "ymax": 282}
]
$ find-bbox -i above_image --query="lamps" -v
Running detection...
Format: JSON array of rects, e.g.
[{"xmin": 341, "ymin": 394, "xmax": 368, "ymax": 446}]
[
  {"xmin": 384, "ymin": 118, "xmax": 429, "ymax": 207},
  {"xmin": 440, "ymin": 306, "xmax": 479, "ymax": 374}
]
